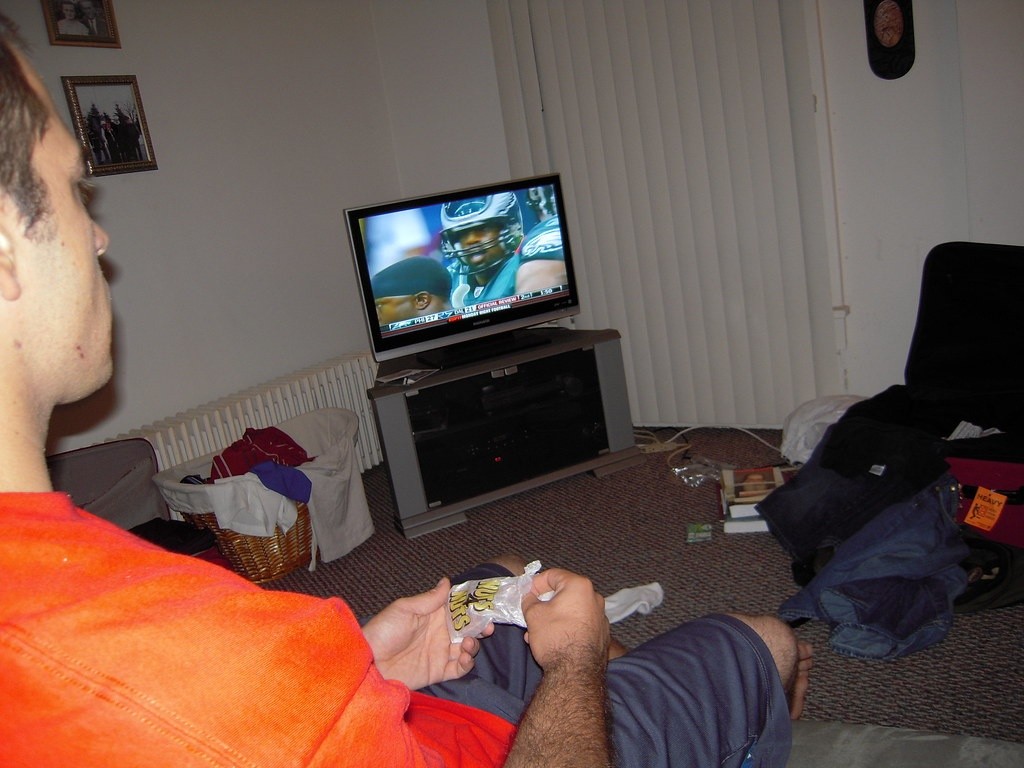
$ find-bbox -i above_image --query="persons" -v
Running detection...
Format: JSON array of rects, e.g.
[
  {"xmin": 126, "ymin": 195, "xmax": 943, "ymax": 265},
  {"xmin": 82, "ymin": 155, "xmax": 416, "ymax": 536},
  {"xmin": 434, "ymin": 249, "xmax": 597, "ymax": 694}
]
[
  {"xmin": 99, "ymin": 116, "xmax": 144, "ymax": 163},
  {"xmin": 513, "ymin": 185, "xmax": 567, "ymax": 297},
  {"xmin": 370, "ymin": 256, "xmax": 456, "ymax": 327},
  {"xmin": 56, "ymin": 1, "xmax": 108, "ymax": 36},
  {"xmin": 0, "ymin": 16, "xmax": 816, "ymax": 767},
  {"xmin": 440, "ymin": 190, "xmax": 525, "ymax": 309}
]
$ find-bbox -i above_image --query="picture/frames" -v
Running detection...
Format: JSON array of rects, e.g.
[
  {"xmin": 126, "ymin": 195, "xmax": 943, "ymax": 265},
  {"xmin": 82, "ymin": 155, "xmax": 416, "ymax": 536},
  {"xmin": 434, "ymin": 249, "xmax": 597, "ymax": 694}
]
[
  {"xmin": 61, "ymin": 75, "xmax": 159, "ymax": 176},
  {"xmin": 42, "ymin": 0, "xmax": 122, "ymax": 48}
]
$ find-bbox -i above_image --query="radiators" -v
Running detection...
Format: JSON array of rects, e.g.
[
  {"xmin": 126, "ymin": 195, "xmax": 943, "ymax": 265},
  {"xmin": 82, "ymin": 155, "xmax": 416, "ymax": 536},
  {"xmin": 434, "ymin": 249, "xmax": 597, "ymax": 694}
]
[{"xmin": 76, "ymin": 348, "xmax": 385, "ymax": 523}]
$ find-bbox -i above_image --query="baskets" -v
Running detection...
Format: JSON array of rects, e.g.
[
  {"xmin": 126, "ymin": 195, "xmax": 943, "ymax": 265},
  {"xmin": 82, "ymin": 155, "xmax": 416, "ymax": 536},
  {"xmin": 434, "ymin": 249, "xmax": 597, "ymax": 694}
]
[{"xmin": 153, "ymin": 407, "xmax": 360, "ymax": 584}]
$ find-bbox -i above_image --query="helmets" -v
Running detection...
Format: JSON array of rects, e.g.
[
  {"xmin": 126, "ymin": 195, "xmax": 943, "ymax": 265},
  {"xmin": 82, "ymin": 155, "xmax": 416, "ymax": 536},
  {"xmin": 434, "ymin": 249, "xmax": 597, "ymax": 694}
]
[{"xmin": 438, "ymin": 191, "xmax": 525, "ymax": 276}]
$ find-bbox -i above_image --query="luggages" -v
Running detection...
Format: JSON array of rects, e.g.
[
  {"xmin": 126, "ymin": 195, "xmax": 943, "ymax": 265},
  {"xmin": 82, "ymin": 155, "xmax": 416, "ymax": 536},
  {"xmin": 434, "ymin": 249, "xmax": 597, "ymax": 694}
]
[{"xmin": 833, "ymin": 242, "xmax": 1024, "ymax": 551}]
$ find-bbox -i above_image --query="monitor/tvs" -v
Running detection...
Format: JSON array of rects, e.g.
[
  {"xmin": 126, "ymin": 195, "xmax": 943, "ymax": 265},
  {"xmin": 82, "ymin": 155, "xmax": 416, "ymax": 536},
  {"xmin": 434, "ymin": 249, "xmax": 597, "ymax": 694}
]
[{"xmin": 344, "ymin": 172, "xmax": 582, "ymax": 369}]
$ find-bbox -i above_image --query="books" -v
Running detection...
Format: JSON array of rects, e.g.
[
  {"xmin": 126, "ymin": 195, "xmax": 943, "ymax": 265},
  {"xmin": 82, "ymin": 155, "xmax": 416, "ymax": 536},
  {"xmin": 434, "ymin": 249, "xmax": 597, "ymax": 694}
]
[{"xmin": 717, "ymin": 465, "xmax": 799, "ymax": 533}]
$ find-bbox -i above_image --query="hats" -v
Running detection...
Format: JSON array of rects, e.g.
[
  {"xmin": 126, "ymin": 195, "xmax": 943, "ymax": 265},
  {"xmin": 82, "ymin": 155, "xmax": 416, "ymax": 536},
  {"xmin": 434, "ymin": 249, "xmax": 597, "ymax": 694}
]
[{"xmin": 370, "ymin": 255, "xmax": 452, "ymax": 298}]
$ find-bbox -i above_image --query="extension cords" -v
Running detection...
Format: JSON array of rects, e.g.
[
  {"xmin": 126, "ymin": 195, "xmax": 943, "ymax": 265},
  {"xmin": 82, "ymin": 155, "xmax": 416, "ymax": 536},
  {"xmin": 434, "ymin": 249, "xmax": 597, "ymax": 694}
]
[{"xmin": 636, "ymin": 441, "xmax": 678, "ymax": 454}]
